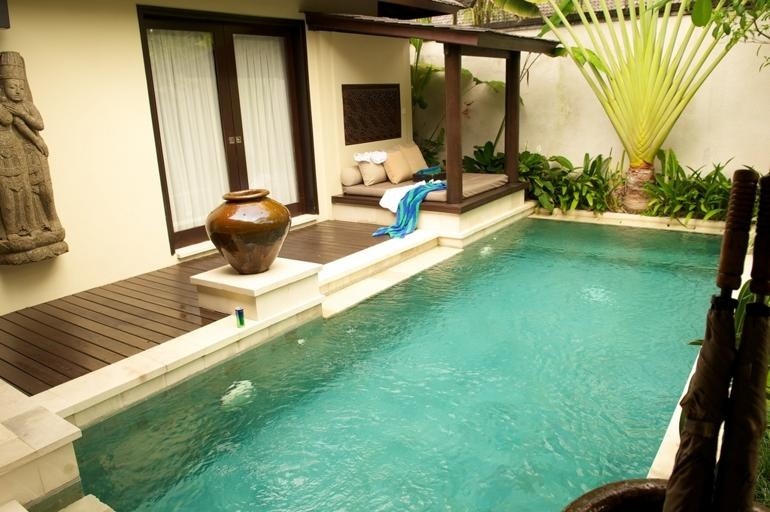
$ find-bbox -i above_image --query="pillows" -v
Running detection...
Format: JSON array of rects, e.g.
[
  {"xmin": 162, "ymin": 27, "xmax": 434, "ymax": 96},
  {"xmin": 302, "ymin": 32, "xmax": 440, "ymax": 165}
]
[{"xmin": 341, "ymin": 144, "xmax": 432, "ymax": 186}]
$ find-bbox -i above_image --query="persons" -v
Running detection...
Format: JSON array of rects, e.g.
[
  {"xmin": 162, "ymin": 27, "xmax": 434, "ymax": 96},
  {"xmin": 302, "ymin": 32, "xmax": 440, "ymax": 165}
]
[{"xmin": 0, "ymin": 50, "xmax": 57, "ymax": 241}]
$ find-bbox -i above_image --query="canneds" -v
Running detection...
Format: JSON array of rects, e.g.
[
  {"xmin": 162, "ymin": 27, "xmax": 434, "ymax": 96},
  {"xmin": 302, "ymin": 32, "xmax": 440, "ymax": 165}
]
[{"xmin": 235, "ymin": 309, "xmax": 244, "ymax": 328}]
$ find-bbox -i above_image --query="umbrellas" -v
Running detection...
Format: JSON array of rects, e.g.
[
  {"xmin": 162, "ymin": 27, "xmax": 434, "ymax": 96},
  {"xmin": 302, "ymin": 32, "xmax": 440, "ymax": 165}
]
[
  {"xmin": 659, "ymin": 168, "xmax": 757, "ymax": 512},
  {"xmin": 711, "ymin": 174, "xmax": 770, "ymax": 511}
]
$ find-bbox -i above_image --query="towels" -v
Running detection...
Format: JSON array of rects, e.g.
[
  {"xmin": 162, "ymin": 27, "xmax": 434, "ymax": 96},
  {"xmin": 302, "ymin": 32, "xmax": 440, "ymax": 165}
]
[{"xmin": 372, "ymin": 180, "xmax": 448, "ymax": 240}]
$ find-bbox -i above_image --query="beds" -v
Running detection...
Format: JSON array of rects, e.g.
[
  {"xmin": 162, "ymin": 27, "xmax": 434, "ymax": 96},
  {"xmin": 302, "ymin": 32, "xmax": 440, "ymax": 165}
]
[{"xmin": 331, "ymin": 167, "xmax": 528, "ymax": 233}]
